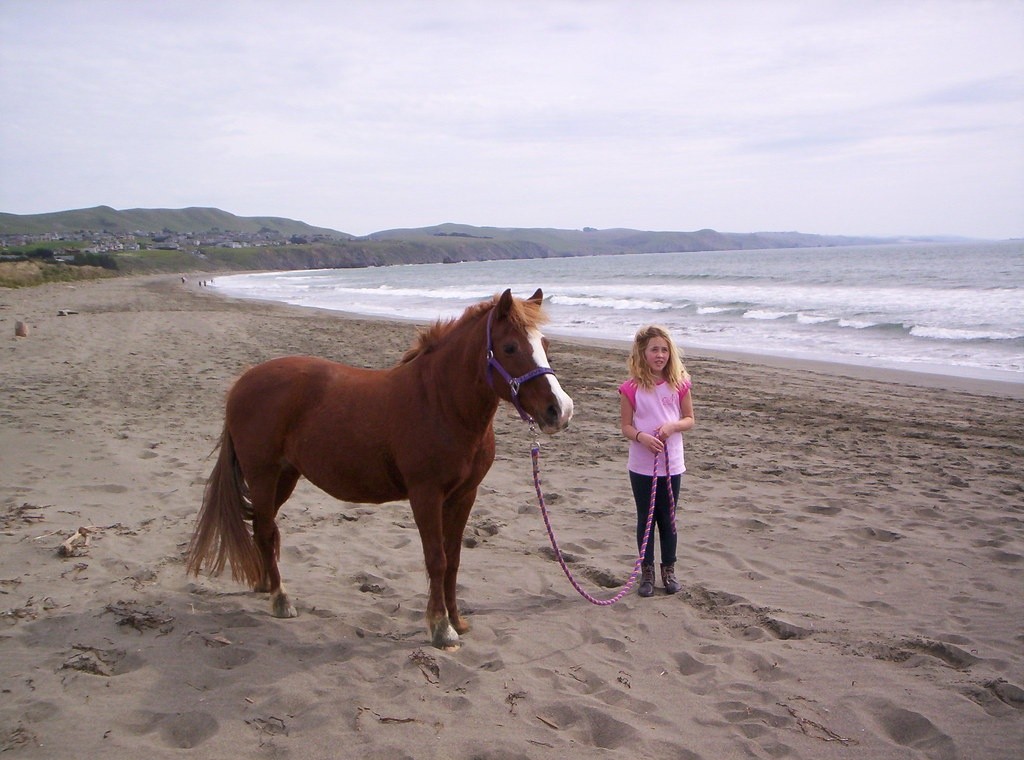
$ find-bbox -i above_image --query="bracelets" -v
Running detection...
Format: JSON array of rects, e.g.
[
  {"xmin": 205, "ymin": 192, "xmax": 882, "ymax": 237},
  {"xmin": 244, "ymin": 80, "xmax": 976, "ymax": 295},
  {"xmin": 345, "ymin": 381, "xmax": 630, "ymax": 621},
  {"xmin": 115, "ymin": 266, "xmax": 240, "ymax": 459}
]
[{"xmin": 636, "ymin": 431, "xmax": 642, "ymax": 442}]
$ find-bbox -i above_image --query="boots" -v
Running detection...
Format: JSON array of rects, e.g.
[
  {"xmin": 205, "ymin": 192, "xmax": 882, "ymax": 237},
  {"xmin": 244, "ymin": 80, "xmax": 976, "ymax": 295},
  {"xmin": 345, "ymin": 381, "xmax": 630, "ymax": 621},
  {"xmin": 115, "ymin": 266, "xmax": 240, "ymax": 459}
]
[
  {"xmin": 638, "ymin": 562, "xmax": 656, "ymax": 597},
  {"xmin": 660, "ymin": 564, "xmax": 682, "ymax": 595}
]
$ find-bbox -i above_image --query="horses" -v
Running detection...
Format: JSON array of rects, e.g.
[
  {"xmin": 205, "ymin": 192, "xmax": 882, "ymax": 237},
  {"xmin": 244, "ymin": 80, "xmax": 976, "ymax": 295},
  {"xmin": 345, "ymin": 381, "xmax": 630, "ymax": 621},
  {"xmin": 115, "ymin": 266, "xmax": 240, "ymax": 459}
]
[{"xmin": 184, "ymin": 287, "xmax": 574, "ymax": 654}]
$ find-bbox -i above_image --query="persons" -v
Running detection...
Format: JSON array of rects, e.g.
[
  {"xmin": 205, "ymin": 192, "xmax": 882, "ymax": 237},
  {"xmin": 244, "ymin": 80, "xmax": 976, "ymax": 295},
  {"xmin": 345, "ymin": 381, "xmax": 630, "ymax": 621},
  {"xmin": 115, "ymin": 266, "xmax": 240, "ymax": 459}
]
[
  {"xmin": 182, "ymin": 276, "xmax": 215, "ymax": 287},
  {"xmin": 617, "ymin": 326, "xmax": 695, "ymax": 596}
]
